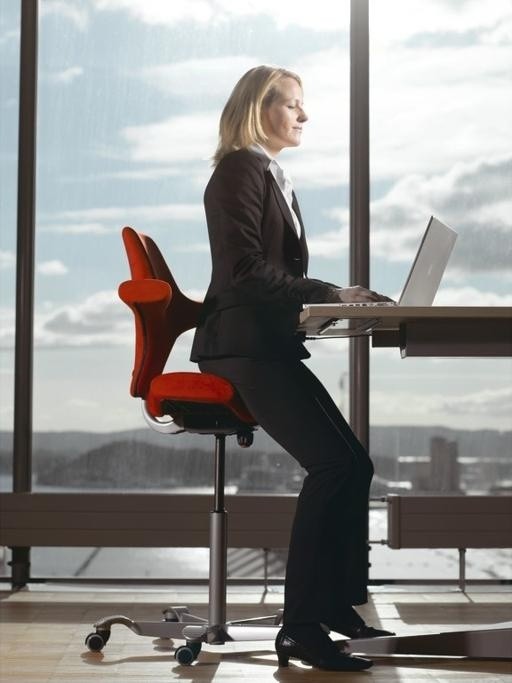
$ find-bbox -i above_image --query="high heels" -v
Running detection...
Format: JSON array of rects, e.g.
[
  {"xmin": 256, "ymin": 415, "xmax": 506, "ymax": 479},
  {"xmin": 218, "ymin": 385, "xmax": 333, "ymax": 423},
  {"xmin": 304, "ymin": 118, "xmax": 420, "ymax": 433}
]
[
  {"xmin": 323, "ymin": 615, "xmax": 395, "ymax": 639},
  {"xmin": 276, "ymin": 628, "xmax": 373, "ymax": 672}
]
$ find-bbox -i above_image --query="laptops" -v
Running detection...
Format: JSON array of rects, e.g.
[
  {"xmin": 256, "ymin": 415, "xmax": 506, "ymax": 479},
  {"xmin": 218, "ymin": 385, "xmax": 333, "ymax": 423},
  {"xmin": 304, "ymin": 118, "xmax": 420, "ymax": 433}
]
[{"xmin": 303, "ymin": 216, "xmax": 457, "ymax": 308}]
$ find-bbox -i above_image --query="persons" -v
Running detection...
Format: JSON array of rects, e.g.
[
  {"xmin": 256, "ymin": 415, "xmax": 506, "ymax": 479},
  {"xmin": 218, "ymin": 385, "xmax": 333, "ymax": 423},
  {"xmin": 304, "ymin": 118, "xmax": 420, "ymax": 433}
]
[{"xmin": 188, "ymin": 64, "xmax": 397, "ymax": 671}]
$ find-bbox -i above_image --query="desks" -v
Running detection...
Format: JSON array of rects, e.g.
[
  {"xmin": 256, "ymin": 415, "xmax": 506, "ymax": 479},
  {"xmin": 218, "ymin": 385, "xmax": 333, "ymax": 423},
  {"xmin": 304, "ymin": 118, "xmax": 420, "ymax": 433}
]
[{"xmin": 299, "ymin": 305, "xmax": 512, "ymax": 659}]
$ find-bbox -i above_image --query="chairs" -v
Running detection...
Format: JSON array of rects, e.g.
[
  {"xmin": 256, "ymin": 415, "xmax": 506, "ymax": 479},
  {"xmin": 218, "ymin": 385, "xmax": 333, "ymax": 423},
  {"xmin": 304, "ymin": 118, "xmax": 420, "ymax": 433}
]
[{"xmin": 85, "ymin": 227, "xmax": 291, "ymax": 668}]
